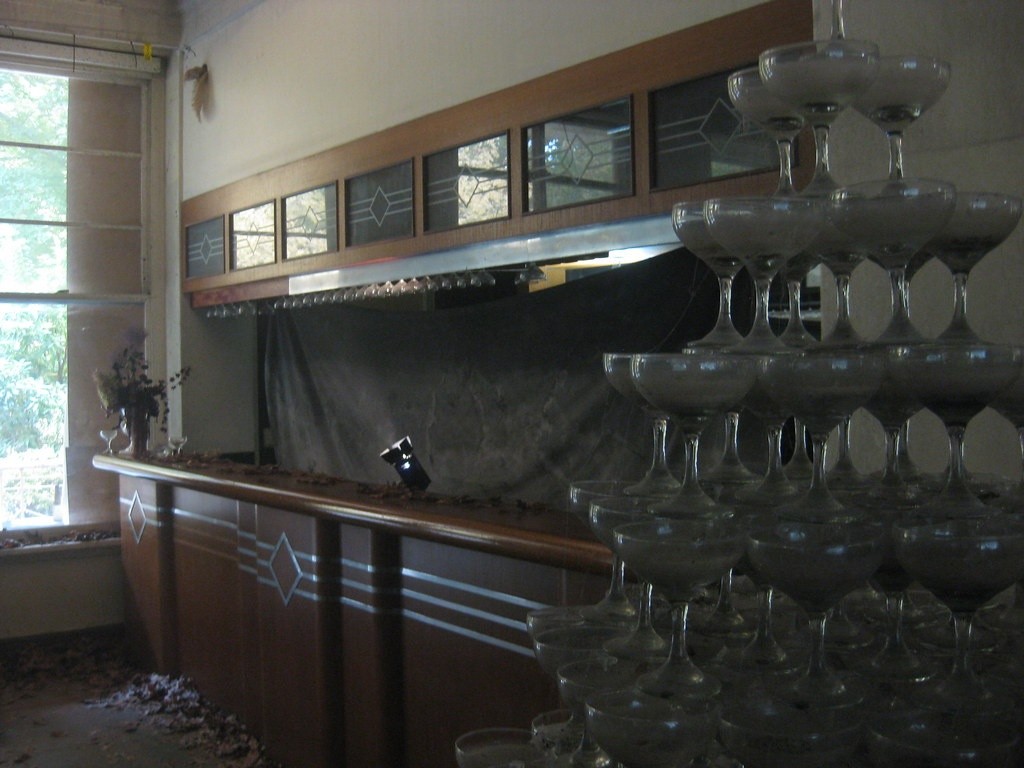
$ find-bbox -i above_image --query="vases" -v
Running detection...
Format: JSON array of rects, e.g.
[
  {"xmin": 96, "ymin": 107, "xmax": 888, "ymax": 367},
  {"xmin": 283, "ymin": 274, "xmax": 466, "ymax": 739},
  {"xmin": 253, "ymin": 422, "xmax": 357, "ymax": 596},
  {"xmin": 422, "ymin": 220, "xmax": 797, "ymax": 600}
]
[{"xmin": 117, "ymin": 403, "xmax": 148, "ymax": 455}]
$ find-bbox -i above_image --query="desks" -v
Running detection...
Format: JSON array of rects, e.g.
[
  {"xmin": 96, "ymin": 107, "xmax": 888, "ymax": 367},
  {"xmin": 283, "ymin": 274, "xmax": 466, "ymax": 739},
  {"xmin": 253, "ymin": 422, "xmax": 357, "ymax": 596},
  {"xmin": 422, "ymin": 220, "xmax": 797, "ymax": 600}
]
[{"xmin": 92, "ymin": 449, "xmax": 612, "ymax": 768}]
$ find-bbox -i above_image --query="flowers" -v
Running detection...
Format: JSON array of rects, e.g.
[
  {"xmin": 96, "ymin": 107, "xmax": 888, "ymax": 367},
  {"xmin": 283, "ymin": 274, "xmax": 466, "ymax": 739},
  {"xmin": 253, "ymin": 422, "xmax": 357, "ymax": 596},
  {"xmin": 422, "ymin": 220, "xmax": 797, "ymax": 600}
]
[{"xmin": 92, "ymin": 326, "xmax": 191, "ymax": 434}]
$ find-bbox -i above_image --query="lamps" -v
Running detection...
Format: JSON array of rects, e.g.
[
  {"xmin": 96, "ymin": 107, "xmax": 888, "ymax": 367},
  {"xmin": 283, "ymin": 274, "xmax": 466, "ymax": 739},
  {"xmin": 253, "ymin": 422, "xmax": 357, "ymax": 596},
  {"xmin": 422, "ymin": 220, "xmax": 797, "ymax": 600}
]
[{"xmin": 380, "ymin": 435, "xmax": 433, "ymax": 495}]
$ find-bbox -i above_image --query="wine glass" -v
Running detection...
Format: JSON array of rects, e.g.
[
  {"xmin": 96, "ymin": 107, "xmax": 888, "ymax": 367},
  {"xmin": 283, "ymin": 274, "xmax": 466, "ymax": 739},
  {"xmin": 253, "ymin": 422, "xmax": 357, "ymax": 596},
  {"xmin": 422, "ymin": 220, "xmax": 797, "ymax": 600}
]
[
  {"xmin": 168, "ymin": 435, "xmax": 187, "ymax": 463},
  {"xmin": 452, "ymin": 2, "xmax": 1024, "ymax": 768},
  {"xmin": 99, "ymin": 430, "xmax": 117, "ymax": 454}
]
